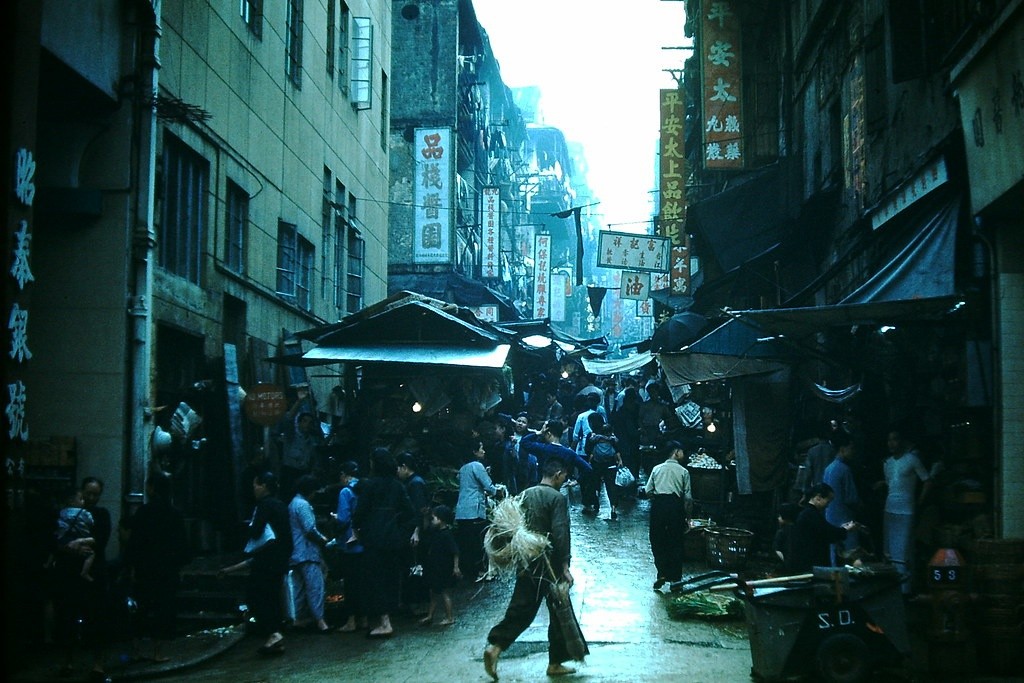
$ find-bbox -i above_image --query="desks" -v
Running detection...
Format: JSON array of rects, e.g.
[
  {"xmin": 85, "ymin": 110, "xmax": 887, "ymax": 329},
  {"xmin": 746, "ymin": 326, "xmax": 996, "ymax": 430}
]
[{"xmin": 686, "ymin": 466, "xmax": 729, "ymax": 526}]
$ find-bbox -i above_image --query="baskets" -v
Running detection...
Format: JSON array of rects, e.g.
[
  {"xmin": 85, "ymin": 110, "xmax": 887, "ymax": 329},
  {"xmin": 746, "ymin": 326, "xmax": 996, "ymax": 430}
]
[
  {"xmin": 704, "ymin": 527, "xmax": 755, "ymax": 571},
  {"xmin": 684, "ymin": 517, "xmax": 718, "ymax": 562},
  {"xmin": 924, "ymin": 536, "xmax": 1024, "ymax": 677}
]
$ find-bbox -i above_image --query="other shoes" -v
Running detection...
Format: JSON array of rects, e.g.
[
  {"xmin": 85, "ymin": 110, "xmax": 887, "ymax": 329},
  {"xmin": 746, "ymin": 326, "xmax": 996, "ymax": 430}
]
[
  {"xmin": 317, "ymin": 620, "xmax": 328, "ymax": 633},
  {"xmin": 671, "ymin": 584, "xmax": 683, "ymax": 591},
  {"xmin": 293, "ymin": 616, "xmax": 312, "ymax": 627},
  {"xmin": 257, "ymin": 630, "xmax": 287, "ymax": 653},
  {"xmin": 368, "ymin": 626, "xmax": 394, "ymax": 637},
  {"xmin": 546, "ymin": 663, "xmax": 576, "ymax": 676},
  {"xmin": 654, "ymin": 576, "xmax": 666, "ymax": 590},
  {"xmin": 440, "ymin": 618, "xmax": 455, "ymax": 625},
  {"xmin": 153, "ymin": 654, "xmax": 170, "ymax": 664},
  {"xmin": 127, "ymin": 652, "xmax": 148, "ymax": 664},
  {"xmin": 64, "ymin": 665, "xmax": 73, "ymax": 677},
  {"xmin": 337, "ymin": 621, "xmax": 368, "ymax": 633},
  {"xmin": 417, "ymin": 615, "xmax": 433, "ymax": 626},
  {"xmin": 90, "ymin": 669, "xmax": 112, "ymax": 682},
  {"xmin": 611, "ymin": 512, "xmax": 618, "ymax": 520},
  {"xmin": 484, "ymin": 643, "xmax": 500, "ymax": 681},
  {"xmin": 581, "ymin": 502, "xmax": 600, "ymax": 514}
]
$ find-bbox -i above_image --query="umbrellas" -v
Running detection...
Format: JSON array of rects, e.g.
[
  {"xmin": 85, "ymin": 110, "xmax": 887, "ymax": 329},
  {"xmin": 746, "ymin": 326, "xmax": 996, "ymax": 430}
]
[{"xmin": 651, "ymin": 311, "xmax": 708, "ymax": 351}]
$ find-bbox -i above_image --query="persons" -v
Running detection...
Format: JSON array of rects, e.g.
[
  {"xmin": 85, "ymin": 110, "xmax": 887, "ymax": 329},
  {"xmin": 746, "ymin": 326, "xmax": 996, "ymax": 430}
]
[
  {"xmin": 52, "ymin": 474, "xmax": 112, "ymax": 646},
  {"xmin": 483, "ymin": 454, "xmax": 574, "ymax": 683},
  {"xmin": 493, "ymin": 365, "xmax": 934, "ymax": 599},
  {"xmin": 225, "ymin": 361, "xmax": 501, "ymax": 655}
]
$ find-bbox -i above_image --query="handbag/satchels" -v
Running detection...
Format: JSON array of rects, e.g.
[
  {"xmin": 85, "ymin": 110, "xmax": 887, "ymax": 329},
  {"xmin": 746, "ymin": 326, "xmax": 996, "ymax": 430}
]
[
  {"xmin": 244, "ymin": 506, "xmax": 275, "ymax": 556},
  {"xmin": 614, "ymin": 467, "xmax": 635, "ymax": 488},
  {"xmin": 484, "ymin": 489, "xmax": 526, "ymax": 565}
]
